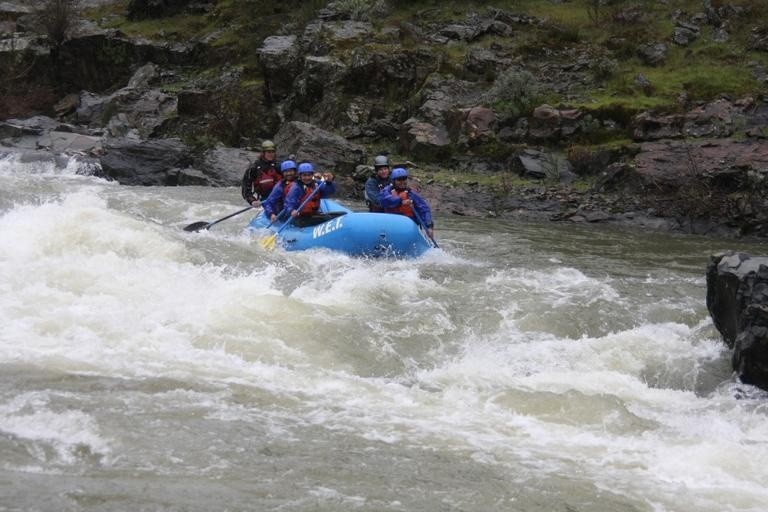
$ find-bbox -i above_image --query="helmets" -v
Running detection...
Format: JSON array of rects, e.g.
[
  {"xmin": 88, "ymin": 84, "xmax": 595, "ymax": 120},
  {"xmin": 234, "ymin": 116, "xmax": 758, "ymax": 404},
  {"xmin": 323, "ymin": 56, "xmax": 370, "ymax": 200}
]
[
  {"xmin": 262, "ymin": 139, "xmax": 276, "ymax": 151},
  {"xmin": 298, "ymin": 163, "xmax": 313, "ymax": 174},
  {"xmin": 391, "ymin": 168, "xmax": 407, "ymax": 179},
  {"xmin": 375, "ymin": 155, "xmax": 389, "ymax": 166},
  {"xmin": 281, "ymin": 160, "xmax": 295, "ymax": 172}
]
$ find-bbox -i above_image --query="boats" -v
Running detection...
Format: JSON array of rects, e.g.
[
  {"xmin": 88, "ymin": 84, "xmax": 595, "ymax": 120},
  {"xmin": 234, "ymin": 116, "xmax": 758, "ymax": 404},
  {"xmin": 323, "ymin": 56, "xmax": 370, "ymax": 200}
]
[{"xmin": 246, "ymin": 200, "xmax": 431, "ymax": 262}]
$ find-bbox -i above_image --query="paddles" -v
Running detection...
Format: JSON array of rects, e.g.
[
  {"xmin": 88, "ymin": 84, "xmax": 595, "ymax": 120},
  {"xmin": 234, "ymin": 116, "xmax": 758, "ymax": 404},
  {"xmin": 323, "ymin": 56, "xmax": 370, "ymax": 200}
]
[
  {"xmin": 183, "ymin": 200, "xmax": 265, "ymax": 232},
  {"xmin": 261, "ymin": 180, "xmax": 325, "ymax": 250}
]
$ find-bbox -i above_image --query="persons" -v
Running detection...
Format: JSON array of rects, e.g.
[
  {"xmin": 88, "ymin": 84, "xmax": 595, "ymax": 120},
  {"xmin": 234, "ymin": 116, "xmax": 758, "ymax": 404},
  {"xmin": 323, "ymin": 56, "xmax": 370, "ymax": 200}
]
[
  {"xmin": 284, "ymin": 162, "xmax": 347, "ymax": 227},
  {"xmin": 265, "ymin": 161, "xmax": 321, "ymax": 224},
  {"xmin": 242, "ymin": 139, "xmax": 296, "ymax": 209},
  {"xmin": 365, "ymin": 155, "xmax": 421, "ymax": 213},
  {"xmin": 379, "ymin": 168, "xmax": 433, "ymax": 239}
]
